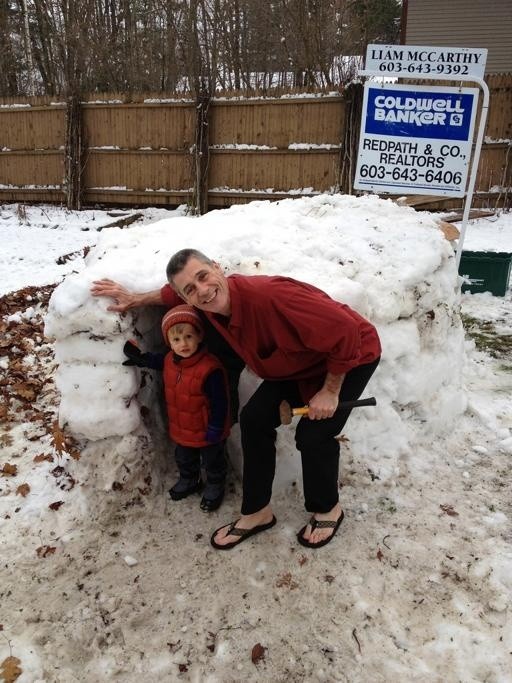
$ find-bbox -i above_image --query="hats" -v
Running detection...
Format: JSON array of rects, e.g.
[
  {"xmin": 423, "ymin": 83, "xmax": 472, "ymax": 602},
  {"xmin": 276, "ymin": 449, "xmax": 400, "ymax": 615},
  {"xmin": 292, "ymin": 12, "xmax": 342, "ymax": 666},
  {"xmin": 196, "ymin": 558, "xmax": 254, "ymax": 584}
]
[{"xmin": 161, "ymin": 304, "xmax": 205, "ymax": 347}]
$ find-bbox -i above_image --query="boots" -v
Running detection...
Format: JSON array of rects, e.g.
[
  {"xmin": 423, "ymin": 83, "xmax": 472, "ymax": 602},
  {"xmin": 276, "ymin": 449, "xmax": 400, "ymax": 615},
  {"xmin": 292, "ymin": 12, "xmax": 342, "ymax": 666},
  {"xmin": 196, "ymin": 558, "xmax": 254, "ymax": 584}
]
[
  {"xmin": 170, "ymin": 466, "xmax": 203, "ymax": 500},
  {"xmin": 201, "ymin": 470, "xmax": 227, "ymax": 510}
]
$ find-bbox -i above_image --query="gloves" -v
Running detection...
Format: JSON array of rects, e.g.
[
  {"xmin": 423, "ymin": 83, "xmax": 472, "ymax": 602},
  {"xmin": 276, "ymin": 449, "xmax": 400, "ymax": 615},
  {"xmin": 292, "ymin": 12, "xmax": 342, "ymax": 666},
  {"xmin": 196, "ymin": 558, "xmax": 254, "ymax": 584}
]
[{"xmin": 122, "ymin": 341, "xmax": 153, "ymax": 367}]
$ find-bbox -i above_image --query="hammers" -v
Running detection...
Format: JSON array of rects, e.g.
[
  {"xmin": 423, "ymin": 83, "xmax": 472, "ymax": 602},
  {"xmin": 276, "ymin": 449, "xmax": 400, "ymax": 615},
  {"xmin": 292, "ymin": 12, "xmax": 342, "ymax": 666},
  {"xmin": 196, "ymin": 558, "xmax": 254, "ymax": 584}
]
[{"xmin": 279, "ymin": 397, "xmax": 377, "ymax": 425}]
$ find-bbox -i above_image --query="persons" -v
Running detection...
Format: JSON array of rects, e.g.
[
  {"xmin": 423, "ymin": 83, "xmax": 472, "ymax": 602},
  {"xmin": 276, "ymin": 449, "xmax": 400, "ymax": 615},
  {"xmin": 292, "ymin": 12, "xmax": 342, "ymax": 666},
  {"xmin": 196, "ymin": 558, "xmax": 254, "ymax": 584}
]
[
  {"xmin": 120, "ymin": 301, "xmax": 233, "ymax": 513},
  {"xmin": 87, "ymin": 246, "xmax": 384, "ymax": 551}
]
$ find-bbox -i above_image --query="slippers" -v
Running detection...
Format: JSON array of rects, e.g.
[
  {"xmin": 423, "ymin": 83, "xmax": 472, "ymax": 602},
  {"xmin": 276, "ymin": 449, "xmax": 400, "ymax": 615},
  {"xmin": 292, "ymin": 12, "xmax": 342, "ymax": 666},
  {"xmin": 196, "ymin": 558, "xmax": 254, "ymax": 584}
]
[
  {"xmin": 298, "ymin": 509, "xmax": 344, "ymax": 548},
  {"xmin": 211, "ymin": 514, "xmax": 276, "ymax": 549}
]
